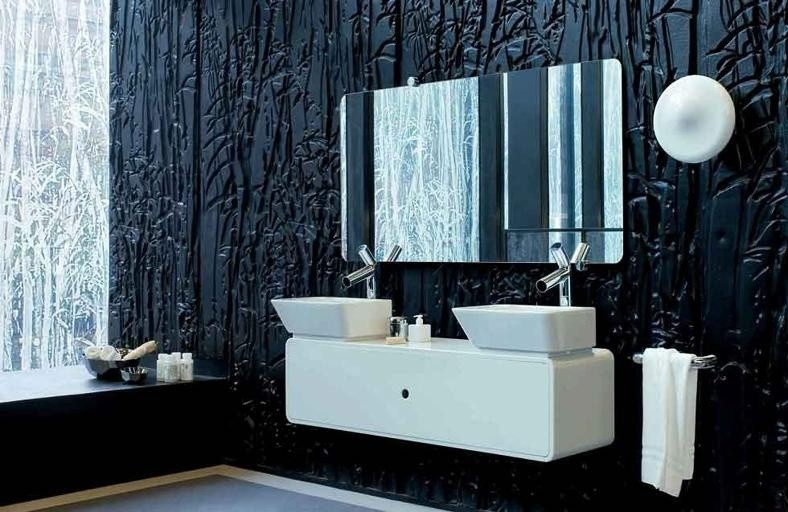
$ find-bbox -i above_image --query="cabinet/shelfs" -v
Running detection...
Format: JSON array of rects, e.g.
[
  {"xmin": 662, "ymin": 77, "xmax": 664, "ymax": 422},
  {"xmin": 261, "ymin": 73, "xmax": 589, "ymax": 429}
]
[{"xmin": 285, "ymin": 335, "xmax": 615, "ymax": 453}]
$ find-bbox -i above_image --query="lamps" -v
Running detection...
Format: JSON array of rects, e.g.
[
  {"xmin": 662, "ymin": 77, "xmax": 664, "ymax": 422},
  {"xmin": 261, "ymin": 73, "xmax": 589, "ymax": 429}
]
[{"xmin": 650, "ymin": 73, "xmax": 734, "ymax": 167}]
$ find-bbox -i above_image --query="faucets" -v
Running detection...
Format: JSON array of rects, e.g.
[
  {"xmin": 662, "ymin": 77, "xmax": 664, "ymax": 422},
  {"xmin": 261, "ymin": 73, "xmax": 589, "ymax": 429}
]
[
  {"xmin": 341, "ymin": 245, "xmax": 404, "ymax": 295},
  {"xmin": 536, "ymin": 240, "xmax": 591, "ymax": 302}
]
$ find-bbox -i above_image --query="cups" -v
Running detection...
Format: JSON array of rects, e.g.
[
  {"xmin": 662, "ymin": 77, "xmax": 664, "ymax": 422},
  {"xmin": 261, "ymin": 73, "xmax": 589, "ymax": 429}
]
[{"xmin": 386, "ymin": 316, "xmax": 408, "ymax": 339}]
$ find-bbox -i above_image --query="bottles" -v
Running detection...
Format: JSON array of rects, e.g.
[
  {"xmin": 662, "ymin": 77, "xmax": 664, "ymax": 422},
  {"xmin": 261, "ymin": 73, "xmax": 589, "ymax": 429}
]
[{"xmin": 156, "ymin": 352, "xmax": 195, "ymax": 385}]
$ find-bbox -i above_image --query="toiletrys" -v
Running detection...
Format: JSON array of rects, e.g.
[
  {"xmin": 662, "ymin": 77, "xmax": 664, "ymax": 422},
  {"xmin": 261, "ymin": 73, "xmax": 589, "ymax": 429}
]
[{"xmin": 408, "ymin": 315, "xmax": 432, "ymax": 343}]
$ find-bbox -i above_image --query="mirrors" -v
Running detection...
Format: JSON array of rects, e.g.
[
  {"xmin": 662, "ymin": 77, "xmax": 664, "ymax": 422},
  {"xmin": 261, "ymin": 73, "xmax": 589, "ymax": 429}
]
[{"xmin": 337, "ymin": 57, "xmax": 624, "ymax": 273}]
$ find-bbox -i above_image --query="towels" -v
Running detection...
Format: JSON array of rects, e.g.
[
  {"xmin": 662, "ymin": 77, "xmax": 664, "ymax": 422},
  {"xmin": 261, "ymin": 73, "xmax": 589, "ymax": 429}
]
[
  {"xmin": 122, "ymin": 340, "xmax": 157, "ymax": 361},
  {"xmin": 99, "ymin": 346, "xmax": 122, "ymax": 360},
  {"xmin": 85, "ymin": 346, "xmax": 100, "ymax": 360},
  {"xmin": 640, "ymin": 347, "xmax": 700, "ymax": 501}
]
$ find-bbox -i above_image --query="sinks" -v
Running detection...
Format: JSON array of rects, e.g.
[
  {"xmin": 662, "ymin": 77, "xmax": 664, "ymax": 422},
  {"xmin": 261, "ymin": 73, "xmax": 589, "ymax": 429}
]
[
  {"xmin": 271, "ymin": 295, "xmax": 393, "ymax": 343},
  {"xmin": 450, "ymin": 304, "xmax": 597, "ymax": 360}
]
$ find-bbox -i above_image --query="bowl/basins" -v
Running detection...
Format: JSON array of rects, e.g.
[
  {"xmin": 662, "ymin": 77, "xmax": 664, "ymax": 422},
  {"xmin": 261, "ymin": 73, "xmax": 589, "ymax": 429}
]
[
  {"xmin": 120, "ymin": 366, "xmax": 147, "ymax": 383},
  {"xmin": 82, "ymin": 348, "xmax": 141, "ymax": 382}
]
